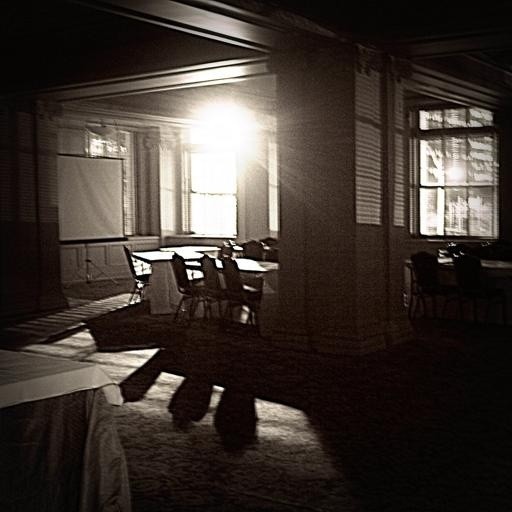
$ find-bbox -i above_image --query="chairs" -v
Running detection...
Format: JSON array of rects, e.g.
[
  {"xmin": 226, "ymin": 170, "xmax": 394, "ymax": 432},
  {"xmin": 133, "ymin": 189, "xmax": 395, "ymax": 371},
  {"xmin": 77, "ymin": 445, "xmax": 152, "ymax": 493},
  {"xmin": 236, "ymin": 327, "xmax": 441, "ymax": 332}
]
[
  {"xmin": 404, "ymin": 238, "xmax": 511, "ymax": 327},
  {"xmin": 122, "ymin": 237, "xmax": 279, "ymax": 334}
]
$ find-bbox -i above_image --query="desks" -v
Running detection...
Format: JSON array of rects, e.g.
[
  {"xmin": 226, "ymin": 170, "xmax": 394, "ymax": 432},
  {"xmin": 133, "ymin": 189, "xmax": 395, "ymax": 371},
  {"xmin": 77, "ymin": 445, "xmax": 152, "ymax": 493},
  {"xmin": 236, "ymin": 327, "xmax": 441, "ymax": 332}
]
[{"xmin": 0, "ymin": 343, "xmax": 136, "ymax": 512}]
